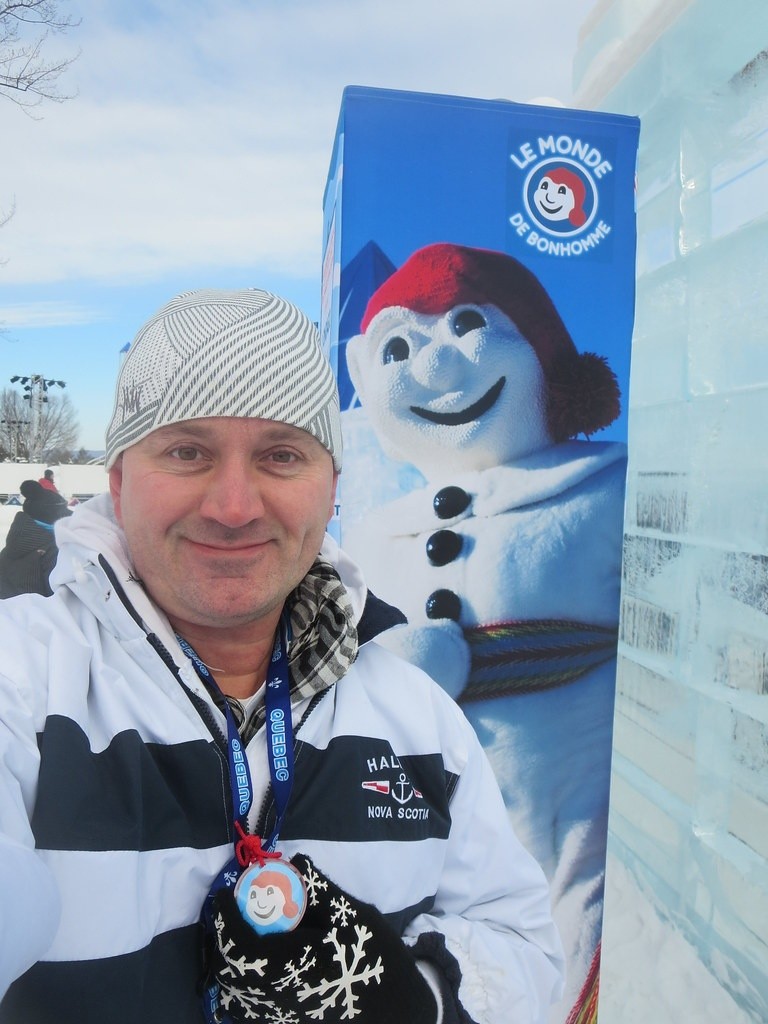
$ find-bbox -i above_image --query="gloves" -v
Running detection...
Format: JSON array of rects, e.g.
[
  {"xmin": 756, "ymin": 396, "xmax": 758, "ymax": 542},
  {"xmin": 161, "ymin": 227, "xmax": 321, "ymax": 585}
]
[{"xmin": 198, "ymin": 851, "xmax": 462, "ymax": 1024}]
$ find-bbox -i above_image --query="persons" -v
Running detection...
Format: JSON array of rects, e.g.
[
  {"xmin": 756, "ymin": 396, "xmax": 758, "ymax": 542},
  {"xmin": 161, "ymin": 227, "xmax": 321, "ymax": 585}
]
[
  {"xmin": 38, "ymin": 469, "xmax": 58, "ymax": 493},
  {"xmin": 0, "ymin": 289, "xmax": 566, "ymax": 1024},
  {"xmin": 0, "ymin": 479, "xmax": 68, "ymax": 599}
]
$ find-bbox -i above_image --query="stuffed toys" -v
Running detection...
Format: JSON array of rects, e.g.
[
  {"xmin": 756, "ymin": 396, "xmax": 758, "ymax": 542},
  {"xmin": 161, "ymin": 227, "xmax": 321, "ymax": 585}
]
[{"xmin": 341, "ymin": 242, "xmax": 627, "ymax": 1024}]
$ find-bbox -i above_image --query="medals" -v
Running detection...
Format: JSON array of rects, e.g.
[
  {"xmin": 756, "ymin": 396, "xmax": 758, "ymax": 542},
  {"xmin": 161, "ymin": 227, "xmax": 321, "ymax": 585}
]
[{"xmin": 233, "ymin": 856, "xmax": 307, "ymax": 937}]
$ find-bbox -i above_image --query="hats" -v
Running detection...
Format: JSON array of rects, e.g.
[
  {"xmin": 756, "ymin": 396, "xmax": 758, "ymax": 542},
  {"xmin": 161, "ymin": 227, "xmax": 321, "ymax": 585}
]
[
  {"xmin": 20, "ymin": 479, "xmax": 73, "ymax": 525},
  {"xmin": 44, "ymin": 470, "xmax": 53, "ymax": 477},
  {"xmin": 105, "ymin": 287, "xmax": 343, "ymax": 477}
]
